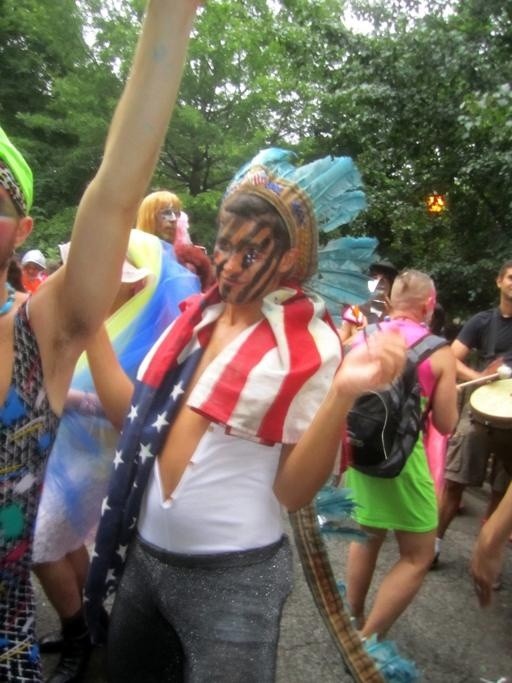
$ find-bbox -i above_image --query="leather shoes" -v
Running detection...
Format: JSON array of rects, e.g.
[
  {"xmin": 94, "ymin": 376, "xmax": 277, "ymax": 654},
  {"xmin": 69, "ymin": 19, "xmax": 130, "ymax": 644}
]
[{"xmin": 36, "ymin": 607, "xmax": 107, "ymax": 683}]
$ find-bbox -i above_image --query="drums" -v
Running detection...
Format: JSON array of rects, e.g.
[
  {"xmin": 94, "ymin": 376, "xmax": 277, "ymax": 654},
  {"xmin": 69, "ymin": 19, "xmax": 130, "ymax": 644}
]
[{"xmin": 469, "ymin": 377, "xmax": 512, "ymax": 429}]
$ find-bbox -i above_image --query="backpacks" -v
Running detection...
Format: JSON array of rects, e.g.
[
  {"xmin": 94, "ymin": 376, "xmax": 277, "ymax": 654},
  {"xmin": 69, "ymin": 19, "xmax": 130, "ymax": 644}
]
[{"xmin": 342, "ymin": 331, "xmax": 451, "ymax": 478}]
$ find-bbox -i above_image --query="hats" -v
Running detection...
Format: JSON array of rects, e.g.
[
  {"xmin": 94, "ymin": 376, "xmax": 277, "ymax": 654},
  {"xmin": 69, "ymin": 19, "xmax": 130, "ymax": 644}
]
[{"xmin": 0, "ymin": 124, "xmax": 34, "ymax": 215}]
